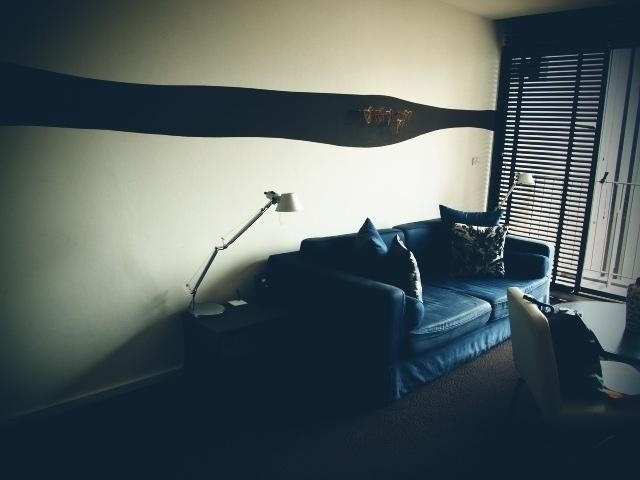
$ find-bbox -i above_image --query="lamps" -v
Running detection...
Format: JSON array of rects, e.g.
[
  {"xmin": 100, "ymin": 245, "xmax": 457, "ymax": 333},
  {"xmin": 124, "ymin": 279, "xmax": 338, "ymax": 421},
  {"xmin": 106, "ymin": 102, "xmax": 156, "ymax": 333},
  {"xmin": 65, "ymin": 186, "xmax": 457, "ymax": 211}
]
[
  {"xmin": 495, "ymin": 171, "xmax": 537, "ymax": 210},
  {"xmin": 185, "ymin": 189, "xmax": 300, "ymax": 318}
]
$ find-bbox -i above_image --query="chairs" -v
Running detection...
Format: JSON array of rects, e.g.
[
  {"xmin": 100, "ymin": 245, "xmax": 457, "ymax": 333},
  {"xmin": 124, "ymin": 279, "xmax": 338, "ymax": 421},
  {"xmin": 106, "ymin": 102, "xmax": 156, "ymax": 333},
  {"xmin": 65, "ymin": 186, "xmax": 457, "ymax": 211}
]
[{"xmin": 506, "ymin": 285, "xmax": 639, "ymax": 456}]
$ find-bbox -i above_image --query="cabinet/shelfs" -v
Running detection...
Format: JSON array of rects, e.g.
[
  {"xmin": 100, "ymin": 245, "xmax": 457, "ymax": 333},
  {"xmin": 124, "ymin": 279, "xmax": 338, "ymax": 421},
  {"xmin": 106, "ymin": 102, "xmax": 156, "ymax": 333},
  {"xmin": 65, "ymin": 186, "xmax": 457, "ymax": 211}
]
[{"xmin": 181, "ymin": 302, "xmax": 297, "ymax": 421}]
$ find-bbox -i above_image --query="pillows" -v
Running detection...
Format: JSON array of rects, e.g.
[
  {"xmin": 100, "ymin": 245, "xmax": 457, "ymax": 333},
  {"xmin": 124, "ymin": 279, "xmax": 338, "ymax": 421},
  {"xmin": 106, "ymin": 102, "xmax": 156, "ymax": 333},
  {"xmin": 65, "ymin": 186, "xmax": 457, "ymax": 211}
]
[
  {"xmin": 354, "ymin": 218, "xmax": 425, "ymax": 332},
  {"xmin": 437, "ymin": 203, "xmax": 512, "ymax": 281}
]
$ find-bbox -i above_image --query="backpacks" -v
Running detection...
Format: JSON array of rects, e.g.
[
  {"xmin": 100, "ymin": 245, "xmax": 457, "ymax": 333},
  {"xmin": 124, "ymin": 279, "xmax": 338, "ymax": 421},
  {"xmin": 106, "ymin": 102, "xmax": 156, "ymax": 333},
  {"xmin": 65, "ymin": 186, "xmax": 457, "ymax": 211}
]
[{"xmin": 543, "ymin": 304, "xmax": 604, "ymax": 403}]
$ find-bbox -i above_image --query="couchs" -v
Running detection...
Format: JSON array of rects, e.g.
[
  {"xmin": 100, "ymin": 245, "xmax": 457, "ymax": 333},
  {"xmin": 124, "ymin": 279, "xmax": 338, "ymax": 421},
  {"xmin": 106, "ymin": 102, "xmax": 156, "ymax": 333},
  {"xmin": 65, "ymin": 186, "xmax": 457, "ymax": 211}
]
[{"xmin": 266, "ymin": 218, "xmax": 556, "ymax": 421}]
